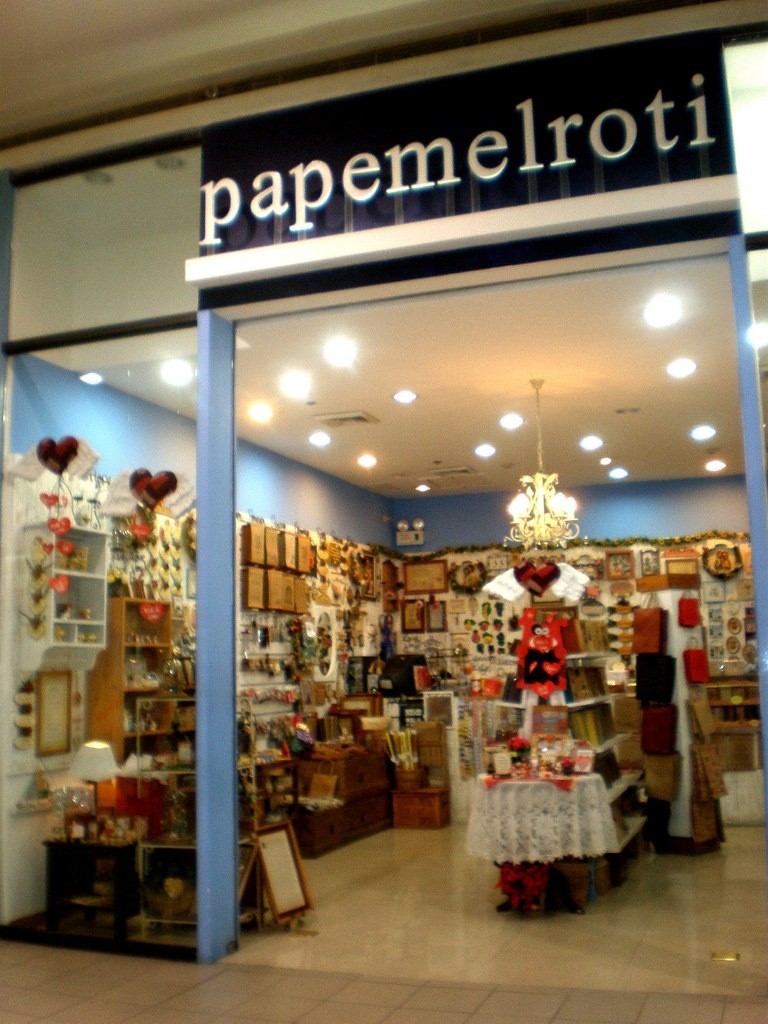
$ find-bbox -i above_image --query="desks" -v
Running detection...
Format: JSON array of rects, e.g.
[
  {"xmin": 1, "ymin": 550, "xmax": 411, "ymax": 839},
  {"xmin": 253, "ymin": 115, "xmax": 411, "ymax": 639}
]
[{"xmin": 464, "ymin": 773, "xmax": 622, "ymax": 916}]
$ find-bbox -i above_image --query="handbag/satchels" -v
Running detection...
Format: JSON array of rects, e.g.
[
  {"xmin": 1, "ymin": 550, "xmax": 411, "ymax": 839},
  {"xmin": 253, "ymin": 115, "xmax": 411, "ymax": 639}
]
[
  {"xmin": 690, "ymin": 738, "xmax": 727, "ymax": 801},
  {"xmin": 631, "ymin": 592, "xmax": 666, "ymax": 654},
  {"xmin": 641, "ymin": 705, "xmax": 676, "ymax": 753},
  {"xmin": 645, "ymin": 752, "xmax": 681, "ymax": 800},
  {"xmin": 688, "ymin": 686, "xmax": 716, "ymax": 737},
  {"xmin": 679, "ymin": 589, "xmax": 700, "ymax": 627},
  {"xmin": 683, "ymin": 638, "xmax": 710, "ymax": 684},
  {"xmin": 636, "ymin": 655, "xmax": 676, "ymax": 703},
  {"xmin": 691, "ymin": 801, "xmax": 717, "ymax": 843}
]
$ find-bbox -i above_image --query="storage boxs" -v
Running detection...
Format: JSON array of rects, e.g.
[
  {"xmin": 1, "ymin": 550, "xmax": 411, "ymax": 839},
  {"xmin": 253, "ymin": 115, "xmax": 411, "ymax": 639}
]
[{"xmin": 292, "ymin": 750, "xmax": 452, "ymax": 860}]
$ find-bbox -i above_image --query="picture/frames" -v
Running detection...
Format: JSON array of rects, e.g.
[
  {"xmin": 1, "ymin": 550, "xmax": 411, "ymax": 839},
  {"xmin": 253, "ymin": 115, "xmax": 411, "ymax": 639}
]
[
  {"xmin": 605, "ymin": 549, "xmax": 635, "ymax": 580},
  {"xmin": 529, "ymin": 733, "xmax": 569, "ymax": 769},
  {"xmin": 339, "ymin": 696, "xmax": 375, "ymax": 751},
  {"xmin": 357, "ymin": 549, "xmax": 450, "ymax": 634},
  {"xmin": 530, "ymin": 554, "xmax": 566, "ymax": 608}
]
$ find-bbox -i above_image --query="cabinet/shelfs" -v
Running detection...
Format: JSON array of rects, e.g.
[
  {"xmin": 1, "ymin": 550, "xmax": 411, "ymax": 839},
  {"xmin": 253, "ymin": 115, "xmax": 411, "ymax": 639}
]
[
  {"xmin": 494, "ymin": 649, "xmax": 648, "ymax": 886},
  {"xmin": 21, "ymin": 521, "xmax": 260, "ymax": 942}
]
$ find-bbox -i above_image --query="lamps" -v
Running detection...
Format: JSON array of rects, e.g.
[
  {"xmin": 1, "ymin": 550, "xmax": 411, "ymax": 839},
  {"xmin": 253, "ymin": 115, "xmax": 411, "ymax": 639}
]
[
  {"xmin": 69, "ymin": 740, "xmax": 119, "ymax": 812},
  {"xmin": 503, "ymin": 378, "xmax": 589, "ymax": 554}
]
